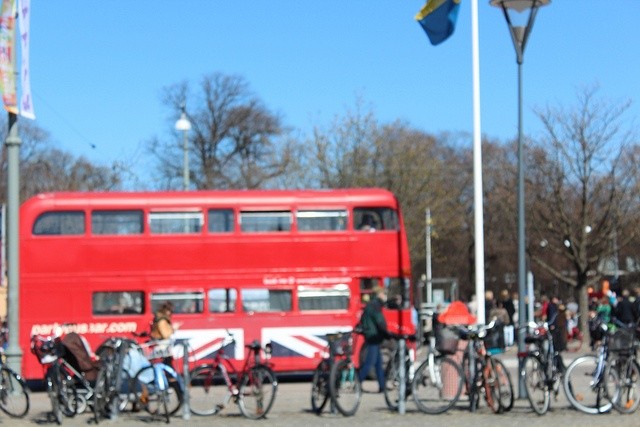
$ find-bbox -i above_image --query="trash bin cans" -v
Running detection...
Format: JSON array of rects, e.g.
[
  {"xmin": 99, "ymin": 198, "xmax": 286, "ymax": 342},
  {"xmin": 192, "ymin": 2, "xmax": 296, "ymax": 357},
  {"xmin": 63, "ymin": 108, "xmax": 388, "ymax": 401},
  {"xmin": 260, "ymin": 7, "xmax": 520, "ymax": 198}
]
[{"xmin": 441, "ymin": 339, "xmax": 484, "ymax": 399}]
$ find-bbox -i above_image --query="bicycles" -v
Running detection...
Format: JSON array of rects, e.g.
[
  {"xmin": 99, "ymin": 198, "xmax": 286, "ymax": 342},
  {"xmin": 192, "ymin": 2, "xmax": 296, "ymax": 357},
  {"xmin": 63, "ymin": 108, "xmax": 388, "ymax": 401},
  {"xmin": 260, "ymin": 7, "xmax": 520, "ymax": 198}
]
[
  {"xmin": 60, "ymin": 358, "xmax": 133, "ymax": 414},
  {"xmin": 383, "ymin": 312, "xmax": 465, "ymax": 414},
  {"xmin": 31, "ymin": 331, "xmax": 77, "ymax": 425},
  {"xmin": 563, "ymin": 310, "xmax": 621, "ymax": 414},
  {"xmin": 477, "ymin": 322, "xmax": 513, "ymax": 414},
  {"xmin": 311, "ymin": 328, "xmax": 364, "ymax": 416},
  {"xmin": 132, "ymin": 331, "xmax": 185, "ymax": 424},
  {"xmin": 0, "ymin": 352, "xmax": 29, "ymax": 418},
  {"xmin": 189, "ymin": 327, "xmax": 278, "ymax": 420},
  {"xmin": 449, "ymin": 315, "xmax": 497, "ymax": 412},
  {"xmin": 515, "ymin": 311, "xmax": 574, "ymax": 415},
  {"xmin": 92, "ymin": 336, "xmax": 138, "ymax": 424},
  {"xmin": 602, "ymin": 317, "xmax": 640, "ymax": 415}
]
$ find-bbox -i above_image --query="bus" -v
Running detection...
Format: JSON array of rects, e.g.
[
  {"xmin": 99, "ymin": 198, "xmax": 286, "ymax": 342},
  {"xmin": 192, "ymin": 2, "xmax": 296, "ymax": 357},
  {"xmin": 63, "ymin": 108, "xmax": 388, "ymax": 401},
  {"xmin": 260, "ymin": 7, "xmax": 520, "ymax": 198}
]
[{"xmin": 22, "ymin": 188, "xmax": 416, "ymax": 391}]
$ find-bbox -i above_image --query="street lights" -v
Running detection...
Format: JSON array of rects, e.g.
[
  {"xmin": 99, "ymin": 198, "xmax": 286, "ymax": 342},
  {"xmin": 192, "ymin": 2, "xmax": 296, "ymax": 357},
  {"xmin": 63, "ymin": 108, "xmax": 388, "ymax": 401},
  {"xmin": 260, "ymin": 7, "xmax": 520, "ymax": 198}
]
[
  {"xmin": 175, "ymin": 107, "xmax": 191, "ymax": 191},
  {"xmin": 489, "ymin": 0, "xmax": 552, "ymax": 401}
]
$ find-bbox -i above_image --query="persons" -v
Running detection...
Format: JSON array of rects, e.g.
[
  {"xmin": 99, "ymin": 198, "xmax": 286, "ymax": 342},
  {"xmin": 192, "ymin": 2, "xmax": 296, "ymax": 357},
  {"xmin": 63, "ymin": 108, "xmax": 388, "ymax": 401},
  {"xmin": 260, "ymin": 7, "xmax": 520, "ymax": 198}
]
[
  {"xmin": 359, "ymin": 215, "xmax": 376, "ymax": 234},
  {"xmin": 391, "ymin": 272, "xmax": 639, "ymax": 354},
  {"xmin": 151, "ymin": 302, "xmax": 189, "ymax": 406},
  {"xmin": 354, "ymin": 286, "xmax": 391, "ymax": 393}
]
